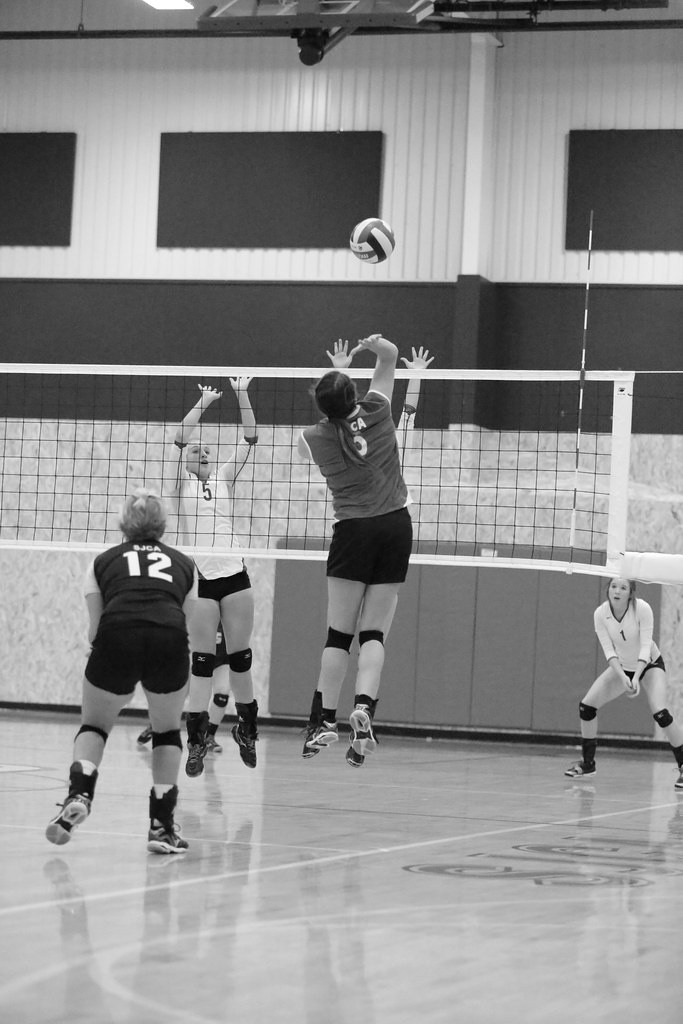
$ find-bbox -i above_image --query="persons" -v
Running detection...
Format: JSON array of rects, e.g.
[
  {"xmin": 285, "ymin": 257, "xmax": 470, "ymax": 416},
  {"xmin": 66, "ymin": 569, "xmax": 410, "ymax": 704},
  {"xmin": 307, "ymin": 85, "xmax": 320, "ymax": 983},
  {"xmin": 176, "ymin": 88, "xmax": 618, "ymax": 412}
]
[
  {"xmin": 138, "ymin": 376, "xmax": 260, "ymax": 775},
  {"xmin": 47, "ymin": 494, "xmax": 201, "ymax": 855},
  {"xmin": 563, "ymin": 577, "xmax": 683, "ymax": 788},
  {"xmin": 300, "ymin": 333, "xmax": 434, "ymax": 766}
]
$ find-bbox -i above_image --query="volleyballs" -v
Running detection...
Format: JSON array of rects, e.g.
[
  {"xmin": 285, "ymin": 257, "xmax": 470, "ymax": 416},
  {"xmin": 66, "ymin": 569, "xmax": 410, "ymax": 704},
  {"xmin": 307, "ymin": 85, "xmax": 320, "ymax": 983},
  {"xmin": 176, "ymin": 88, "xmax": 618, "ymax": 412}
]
[{"xmin": 349, "ymin": 217, "xmax": 395, "ymax": 265}]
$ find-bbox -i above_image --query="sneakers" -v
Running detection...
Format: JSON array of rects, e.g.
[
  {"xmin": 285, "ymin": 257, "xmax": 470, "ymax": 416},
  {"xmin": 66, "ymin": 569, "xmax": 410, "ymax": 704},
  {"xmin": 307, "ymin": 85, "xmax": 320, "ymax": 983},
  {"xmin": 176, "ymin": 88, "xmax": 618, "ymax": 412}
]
[
  {"xmin": 346, "ymin": 745, "xmax": 364, "ymax": 768},
  {"xmin": 146, "ymin": 823, "xmax": 188, "ymax": 853},
  {"xmin": 231, "ymin": 724, "xmax": 259, "ymax": 768},
  {"xmin": 137, "ymin": 724, "xmax": 152, "ymax": 745},
  {"xmin": 46, "ymin": 793, "xmax": 91, "ymax": 845},
  {"xmin": 675, "ymin": 765, "xmax": 683, "ymax": 788},
  {"xmin": 301, "ymin": 723, "xmax": 320, "ymax": 759},
  {"xmin": 306, "ymin": 721, "xmax": 339, "ymax": 749},
  {"xmin": 565, "ymin": 760, "xmax": 596, "ymax": 777},
  {"xmin": 349, "ymin": 704, "xmax": 379, "ymax": 756},
  {"xmin": 203, "ymin": 732, "xmax": 223, "ymax": 753},
  {"xmin": 185, "ymin": 739, "xmax": 207, "ymax": 777}
]
